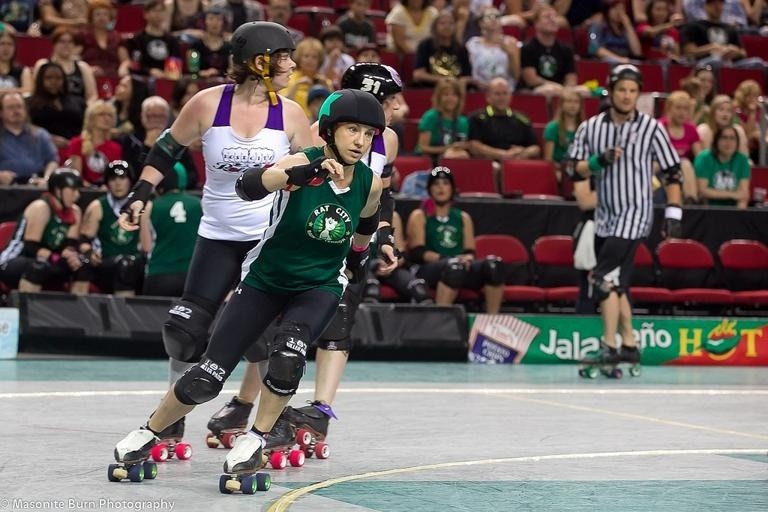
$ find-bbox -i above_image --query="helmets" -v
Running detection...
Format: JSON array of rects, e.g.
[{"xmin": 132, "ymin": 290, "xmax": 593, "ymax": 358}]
[
  {"xmin": 232, "ymin": 20, "xmax": 298, "ymax": 79},
  {"xmin": 103, "ymin": 158, "xmax": 136, "ymax": 184},
  {"xmin": 338, "ymin": 60, "xmax": 405, "ymax": 118},
  {"xmin": 316, "ymin": 87, "xmax": 385, "ymax": 143},
  {"xmin": 153, "ymin": 170, "xmax": 180, "ymax": 193},
  {"xmin": 46, "ymin": 166, "xmax": 84, "ymax": 188},
  {"xmin": 606, "ymin": 63, "xmax": 643, "ymax": 93},
  {"xmin": 427, "ymin": 162, "xmax": 454, "ymax": 194}
]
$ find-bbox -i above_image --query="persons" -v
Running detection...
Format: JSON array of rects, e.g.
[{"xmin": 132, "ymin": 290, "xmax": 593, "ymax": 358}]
[
  {"xmin": 113, "ymin": 86, "xmax": 390, "ymax": 475},
  {"xmin": 1, "ymin": 3, "xmax": 768, "ymax": 316},
  {"xmin": 570, "ymin": 64, "xmax": 685, "ymax": 379},
  {"xmin": 111, "ymin": 19, "xmax": 318, "ymax": 443},
  {"xmin": 207, "ymin": 60, "xmax": 406, "ymax": 442}
]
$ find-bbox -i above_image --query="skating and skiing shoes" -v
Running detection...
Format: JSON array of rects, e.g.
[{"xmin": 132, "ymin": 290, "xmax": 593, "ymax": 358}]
[
  {"xmin": 217, "ymin": 427, "xmax": 272, "ymax": 495},
  {"xmin": 202, "ymin": 395, "xmax": 252, "ymax": 450},
  {"xmin": 262, "ymin": 419, "xmax": 308, "ymax": 468},
  {"xmin": 581, "ymin": 343, "xmax": 625, "ymax": 380},
  {"xmin": 106, "ymin": 420, "xmax": 163, "ymax": 482},
  {"xmin": 152, "ymin": 414, "xmax": 196, "ymax": 463},
  {"xmin": 612, "ymin": 343, "xmax": 645, "ymax": 378},
  {"xmin": 282, "ymin": 399, "xmax": 337, "ymax": 462}
]
